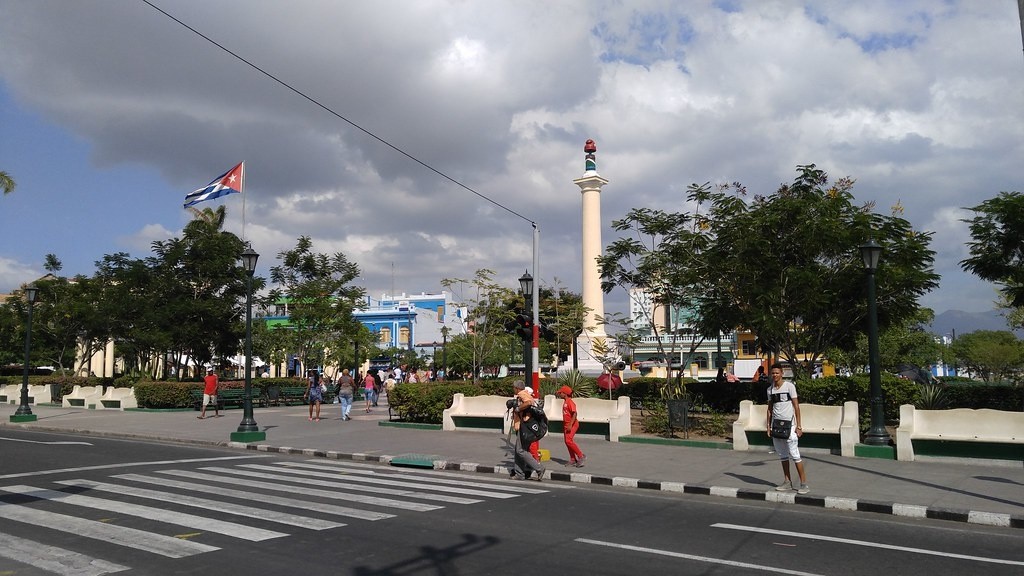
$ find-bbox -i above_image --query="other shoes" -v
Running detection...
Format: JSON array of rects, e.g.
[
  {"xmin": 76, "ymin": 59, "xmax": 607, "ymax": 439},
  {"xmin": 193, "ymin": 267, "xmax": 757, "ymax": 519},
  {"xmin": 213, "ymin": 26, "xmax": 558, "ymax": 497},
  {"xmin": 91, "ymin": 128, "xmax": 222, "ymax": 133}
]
[
  {"xmin": 215, "ymin": 414, "xmax": 219, "ymax": 417},
  {"xmin": 797, "ymin": 482, "xmax": 811, "ymax": 495},
  {"xmin": 565, "ymin": 460, "xmax": 577, "ymax": 467},
  {"xmin": 537, "ymin": 466, "xmax": 546, "ymax": 481},
  {"xmin": 315, "ymin": 418, "xmax": 320, "ymax": 422},
  {"xmin": 774, "ymin": 481, "xmax": 793, "ymax": 491},
  {"xmin": 307, "ymin": 417, "xmax": 313, "ymax": 421},
  {"xmin": 196, "ymin": 416, "xmax": 206, "ymax": 419},
  {"xmin": 345, "ymin": 414, "xmax": 352, "ymax": 419},
  {"xmin": 576, "ymin": 454, "xmax": 588, "ymax": 468},
  {"xmin": 508, "ymin": 474, "xmax": 525, "ymax": 480}
]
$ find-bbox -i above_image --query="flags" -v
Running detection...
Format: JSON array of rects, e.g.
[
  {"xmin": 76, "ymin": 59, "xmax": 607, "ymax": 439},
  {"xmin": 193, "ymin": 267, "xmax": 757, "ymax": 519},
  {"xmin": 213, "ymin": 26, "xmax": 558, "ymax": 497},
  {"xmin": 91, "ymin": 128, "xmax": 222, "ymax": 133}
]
[{"xmin": 183, "ymin": 161, "xmax": 244, "ymax": 209}]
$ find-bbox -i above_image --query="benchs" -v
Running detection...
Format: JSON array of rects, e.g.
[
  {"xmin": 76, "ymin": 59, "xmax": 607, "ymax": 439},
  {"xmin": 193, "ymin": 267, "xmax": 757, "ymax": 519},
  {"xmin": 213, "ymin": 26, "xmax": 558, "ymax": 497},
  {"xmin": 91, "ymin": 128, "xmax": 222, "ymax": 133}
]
[
  {"xmin": 543, "ymin": 394, "xmax": 631, "ymax": 442},
  {"xmin": 895, "ymin": 403, "xmax": 1024, "ymax": 461},
  {"xmin": 63, "ymin": 385, "xmax": 103, "ymax": 409},
  {"xmin": 96, "ymin": 386, "xmax": 139, "ymax": 411},
  {"xmin": 280, "ymin": 386, "xmax": 335, "ymax": 406},
  {"xmin": 0, "ymin": 384, "xmax": 51, "ymax": 406},
  {"xmin": 733, "ymin": 400, "xmax": 861, "ymax": 457},
  {"xmin": 442, "ymin": 393, "xmax": 518, "ymax": 435},
  {"xmin": 193, "ymin": 388, "xmax": 262, "ymax": 410}
]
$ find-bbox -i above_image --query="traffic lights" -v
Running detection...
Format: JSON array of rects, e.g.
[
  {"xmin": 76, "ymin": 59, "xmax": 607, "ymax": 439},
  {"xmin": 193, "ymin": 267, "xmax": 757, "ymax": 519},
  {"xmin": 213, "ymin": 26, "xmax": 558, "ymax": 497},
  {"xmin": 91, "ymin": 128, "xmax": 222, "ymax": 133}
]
[{"xmin": 516, "ymin": 311, "xmax": 533, "ymax": 340}]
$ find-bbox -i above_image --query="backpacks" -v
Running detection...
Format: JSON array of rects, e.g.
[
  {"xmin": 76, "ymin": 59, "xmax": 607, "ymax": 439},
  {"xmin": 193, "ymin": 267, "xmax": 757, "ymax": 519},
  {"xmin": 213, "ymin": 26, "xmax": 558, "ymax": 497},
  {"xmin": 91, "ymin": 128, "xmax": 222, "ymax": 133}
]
[{"xmin": 517, "ymin": 397, "xmax": 549, "ymax": 445}]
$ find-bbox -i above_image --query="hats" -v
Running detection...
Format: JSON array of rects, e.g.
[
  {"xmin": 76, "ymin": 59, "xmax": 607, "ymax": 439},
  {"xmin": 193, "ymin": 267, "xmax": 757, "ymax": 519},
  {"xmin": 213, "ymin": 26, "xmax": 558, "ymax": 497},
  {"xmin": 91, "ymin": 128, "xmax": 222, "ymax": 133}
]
[
  {"xmin": 207, "ymin": 368, "xmax": 213, "ymax": 371},
  {"xmin": 556, "ymin": 385, "xmax": 572, "ymax": 396}
]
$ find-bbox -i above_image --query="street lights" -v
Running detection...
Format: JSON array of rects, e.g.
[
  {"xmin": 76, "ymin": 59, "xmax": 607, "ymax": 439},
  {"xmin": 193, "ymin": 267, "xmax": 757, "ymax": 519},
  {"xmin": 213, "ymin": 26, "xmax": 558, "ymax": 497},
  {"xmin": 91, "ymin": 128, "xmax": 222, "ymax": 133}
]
[
  {"xmin": 518, "ymin": 269, "xmax": 533, "ymax": 389},
  {"xmin": 858, "ymin": 233, "xmax": 895, "ymax": 445},
  {"xmin": 230, "ymin": 243, "xmax": 266, "ymax": 443},
  {"xmin": 10, "ymin": 285, "xmax": 39, "ymax": 422},
  {"xmin": 441, "ymin": 325, "xmax": 448, "ymax": 377},
  {"xmin": 432, "ymin": 341, "xmax": 438, "ymax": 379},
  {"xmin": 350, "ymin": 333, "xmax": 361, "ymax": 396}
]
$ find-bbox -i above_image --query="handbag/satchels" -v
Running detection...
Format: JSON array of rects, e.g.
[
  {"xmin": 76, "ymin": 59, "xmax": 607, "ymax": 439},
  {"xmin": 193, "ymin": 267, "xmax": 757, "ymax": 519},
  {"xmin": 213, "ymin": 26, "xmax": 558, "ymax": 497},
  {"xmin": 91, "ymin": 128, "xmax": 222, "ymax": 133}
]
[
  {"xmin": 771, "ymin": 418, "xmax": 794, "ymax": 439},
  {"xmin": 334, "ymin": 383, "xmax": 342, "ymax": 394},
  {"xmin": 319, "ymin": 383, "xmax": 327, "ymax": 393}
]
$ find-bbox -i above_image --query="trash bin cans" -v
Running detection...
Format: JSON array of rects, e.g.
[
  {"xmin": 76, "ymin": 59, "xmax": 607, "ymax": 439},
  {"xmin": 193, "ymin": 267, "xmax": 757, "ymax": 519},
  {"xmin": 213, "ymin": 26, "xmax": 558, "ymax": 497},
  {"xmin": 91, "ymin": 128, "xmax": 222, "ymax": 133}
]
[
  {"xmin": 267, "ymin": 386, "xmax": 280, "ymax": 400},
  {"xmin": 665, "ymin": 399, "xmax": 689, "ymax": 429},
  {"xmin": 50, "ymin": 384, "xmax": 62, "ymax": 400}
]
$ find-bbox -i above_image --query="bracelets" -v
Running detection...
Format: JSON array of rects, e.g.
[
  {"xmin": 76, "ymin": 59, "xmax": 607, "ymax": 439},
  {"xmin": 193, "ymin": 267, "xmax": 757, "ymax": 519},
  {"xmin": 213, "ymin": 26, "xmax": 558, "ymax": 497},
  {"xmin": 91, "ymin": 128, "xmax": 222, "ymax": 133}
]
[{"xmin": 796, "ymin": 426, "xmax": 804, "ymax": 431}]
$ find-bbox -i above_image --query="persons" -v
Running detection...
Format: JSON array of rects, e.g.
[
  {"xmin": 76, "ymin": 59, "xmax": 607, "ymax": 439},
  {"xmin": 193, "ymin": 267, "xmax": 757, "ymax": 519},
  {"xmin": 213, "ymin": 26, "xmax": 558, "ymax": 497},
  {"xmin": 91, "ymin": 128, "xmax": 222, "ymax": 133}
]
[
  {"xmin": 384, "ymin": 364, "xmax": 474, "ymax": 400},
  {"xmin": 557, "ymin": 385, "xmax": 587, "ymax": 467},
  {"xmin": 767, "ymin": 364, "xmax": 810, "ymax": 494},
  {"xmin": 261, "ymin": 369, "xmax": 269, "ymax": 378},
  {"xmin": 509, "ymin": 380, "xmax": 546, "ymax": 480},
  {"xmin": 923, "ymin": 364, "xmax": 939, "ymax": 386},
  {"xmin": 197, "ymin": 367, "xmax": 219, "ymax": 419},
  {"xmin": 362, "ymin": 368, "xmax": 382, "ymax": 413},
  {"xmin": 758, "ymin": 366, "xmax": 768, "ymax": 382},
  {"xmin": 338, "ymin": 369, "xmax": 356, "ymax": 421},
  {"xmin": 304, "ymin": 371, "xmax": 323, "ymax": 422}
]
